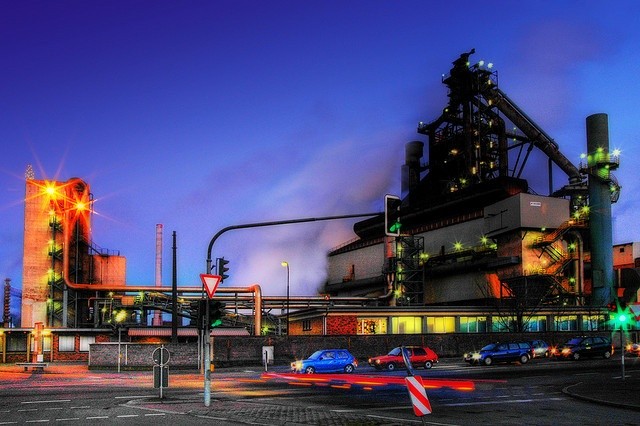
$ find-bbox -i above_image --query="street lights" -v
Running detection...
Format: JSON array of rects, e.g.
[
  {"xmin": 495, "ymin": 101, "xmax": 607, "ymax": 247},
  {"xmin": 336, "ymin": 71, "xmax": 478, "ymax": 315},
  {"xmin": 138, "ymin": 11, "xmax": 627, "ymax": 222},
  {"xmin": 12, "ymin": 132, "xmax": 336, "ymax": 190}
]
[{"xmin": 280, "ymin": 260, "xmax": 291, "ymax": 336}]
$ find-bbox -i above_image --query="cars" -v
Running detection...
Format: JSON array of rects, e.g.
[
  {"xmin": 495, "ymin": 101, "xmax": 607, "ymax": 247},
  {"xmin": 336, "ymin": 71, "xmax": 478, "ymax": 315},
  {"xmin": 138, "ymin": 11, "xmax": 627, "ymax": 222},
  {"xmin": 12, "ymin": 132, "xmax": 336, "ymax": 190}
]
[
  {"xmin": 368, "ymin": 345, "xmax": 439, "ymax": 371},
  {"xmin": 291, "ymin": 348, "xmax": 358, "ymax": 374},
  {"xmin": 627, "ymin": 343, "xmax": 640, "ymax": 357},
  {"xmin": 529, "ymin": 340, "xmax": 551, "ymax": 358},
  {"xmin": 465, "ymin": 340, "xmax": 532, "ymax": 365}
]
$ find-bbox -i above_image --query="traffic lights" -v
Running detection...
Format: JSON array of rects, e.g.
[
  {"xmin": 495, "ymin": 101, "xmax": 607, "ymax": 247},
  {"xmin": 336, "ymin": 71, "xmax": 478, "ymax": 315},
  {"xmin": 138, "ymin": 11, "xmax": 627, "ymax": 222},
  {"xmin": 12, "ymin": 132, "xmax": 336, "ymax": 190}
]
[
  {"xmin": 383, "ymin": 194, "xmax": 402, "ymax": 236},
  {"xmin": 218, "ymin": 258, "xmax": 229, "ymax": 283}
]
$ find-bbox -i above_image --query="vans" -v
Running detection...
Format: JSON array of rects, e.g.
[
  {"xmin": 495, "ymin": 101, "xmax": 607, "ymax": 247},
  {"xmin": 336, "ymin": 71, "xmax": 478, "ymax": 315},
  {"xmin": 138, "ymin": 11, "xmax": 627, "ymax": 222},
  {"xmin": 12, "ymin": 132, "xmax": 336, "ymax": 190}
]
[{"xmin": 552, "ymin": 335, "xmax": 615, "ymax": 359}]
